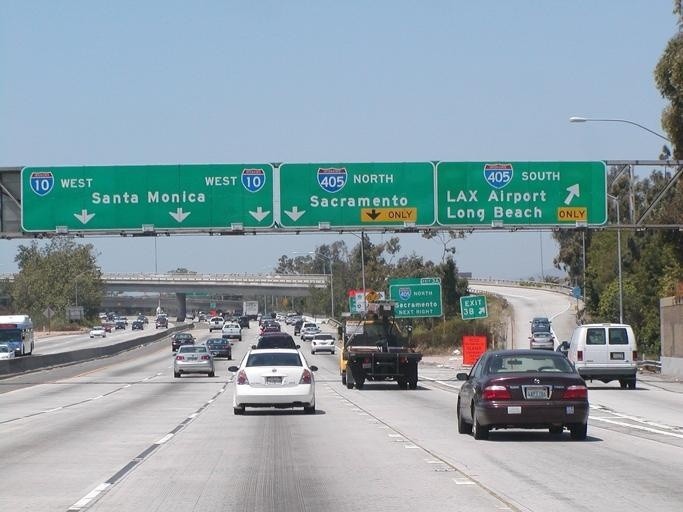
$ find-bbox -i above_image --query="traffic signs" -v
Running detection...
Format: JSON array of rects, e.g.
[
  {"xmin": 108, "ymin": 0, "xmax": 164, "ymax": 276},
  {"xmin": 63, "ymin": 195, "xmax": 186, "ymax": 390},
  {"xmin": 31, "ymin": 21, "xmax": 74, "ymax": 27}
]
[
  {"xmin": 435, "ymin": 160, "xmax": 608, "ymax": 228},
  {"xmin": 459, "ymin": 295, "xmax": 488, "ymax": 321},
  {"xmin": 18, "ymin": 163, "xmax": 272, "ymax": 230},
  {"xmin": 389, "ymin": 277, "xmax": 442, "ymax": 318},
  {"xmin": 279, "ymin": 160, "xmax": 436, "ymax": 231}
]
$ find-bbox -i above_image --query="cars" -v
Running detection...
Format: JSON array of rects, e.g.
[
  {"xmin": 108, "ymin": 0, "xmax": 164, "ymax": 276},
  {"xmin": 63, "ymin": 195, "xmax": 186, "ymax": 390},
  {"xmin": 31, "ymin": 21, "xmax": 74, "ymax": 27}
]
[
  {"xmin": 456, "ymin": 349, "xmax": 588, "ymax": 440},
  {"xmin": 529, "ymin": 318, "xmax": 552, "ymax": 334},
  {"xmin": 0, "ymin": 344, "xmax": 14, "ymax": 360},
  {"xmin": 527, "ymin": 333, "xmax": 555, "ymax": 349},
  {"xmin": 556, "ymin": 343, "xmax": 569, "ymax": 356},
  {"xmin": 87, "ymin": 310, "xmax": 169, "ymax": 338}
]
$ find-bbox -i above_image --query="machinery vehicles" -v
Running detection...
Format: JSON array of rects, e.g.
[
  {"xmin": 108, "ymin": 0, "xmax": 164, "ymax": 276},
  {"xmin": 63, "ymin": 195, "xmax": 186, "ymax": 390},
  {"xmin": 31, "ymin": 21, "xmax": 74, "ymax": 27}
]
[
  {"xmin": 335, "ymin": 303, "xmax": 423, "ymax": 392},
  {"xmin": 171, "ymin": 309, "xmax": 336, "ymax": 416}
]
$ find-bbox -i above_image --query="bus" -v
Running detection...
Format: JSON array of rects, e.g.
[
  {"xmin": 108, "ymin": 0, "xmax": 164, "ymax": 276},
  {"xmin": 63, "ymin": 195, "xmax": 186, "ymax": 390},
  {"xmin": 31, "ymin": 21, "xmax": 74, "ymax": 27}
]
[{"xmin": 0, "ymin": 315, "xmax": 34, "ymax": 355}]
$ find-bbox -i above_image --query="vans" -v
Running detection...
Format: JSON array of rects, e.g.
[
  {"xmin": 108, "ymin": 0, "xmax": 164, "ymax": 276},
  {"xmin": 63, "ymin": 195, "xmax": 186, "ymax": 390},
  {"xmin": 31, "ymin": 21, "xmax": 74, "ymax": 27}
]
[{"xmin": 562, "ymin": 323, "xmax": 639, "ymax": 390}]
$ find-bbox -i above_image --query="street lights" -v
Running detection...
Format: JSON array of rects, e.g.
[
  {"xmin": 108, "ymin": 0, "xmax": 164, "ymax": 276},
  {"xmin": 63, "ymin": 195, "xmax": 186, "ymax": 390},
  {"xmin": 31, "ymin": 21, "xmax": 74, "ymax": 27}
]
[
  {"xmin": 566, "ymin": 115, "xmax": 675, "ymax": 146},
  {"xmin": 74, "ymin": 267, "xmax": 101, "ymax": 306},
  {"xmin": 292, "ymin": 251, "xmax": 327, "ymax": 275},
  {"xmin": 308, "ymin": 251, "xmax": 335, "ymax": 316}
]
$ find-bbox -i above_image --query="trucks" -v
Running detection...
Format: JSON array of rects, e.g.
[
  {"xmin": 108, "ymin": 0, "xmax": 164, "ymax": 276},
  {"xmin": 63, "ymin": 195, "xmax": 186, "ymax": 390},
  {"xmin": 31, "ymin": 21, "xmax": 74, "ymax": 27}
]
[{"xmin": 241, "ymin": 301, "xmax": 258, "ymax": 320}]
